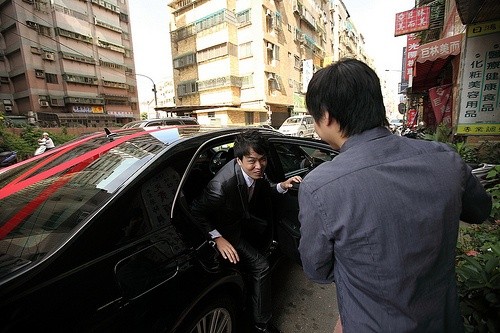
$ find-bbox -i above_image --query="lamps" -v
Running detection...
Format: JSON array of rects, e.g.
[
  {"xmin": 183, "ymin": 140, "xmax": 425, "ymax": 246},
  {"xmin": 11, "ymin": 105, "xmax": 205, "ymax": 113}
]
[{"xmin": 37, "ymin": 72, "xmax": 45, "ymax": 77}]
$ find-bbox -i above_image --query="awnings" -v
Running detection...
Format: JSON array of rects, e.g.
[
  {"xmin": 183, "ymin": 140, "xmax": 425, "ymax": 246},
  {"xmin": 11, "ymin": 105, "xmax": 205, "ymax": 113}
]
[{"xmin": 412, "ymin": 32, "xmax": 464, "ymax": 78}]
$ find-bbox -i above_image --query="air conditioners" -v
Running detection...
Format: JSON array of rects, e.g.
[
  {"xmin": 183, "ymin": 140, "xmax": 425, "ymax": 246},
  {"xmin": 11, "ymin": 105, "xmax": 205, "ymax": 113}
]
[
  {"xmin": 46, "ymin": 52, "xmax": 54, "ymax": 59},
  {"xmin": 41, "ymin": 101, "xmax": 48, "ymax": 107},
  {"xmin": 5, "ymin": 106, "xmax": 12, "ymax": 111}
]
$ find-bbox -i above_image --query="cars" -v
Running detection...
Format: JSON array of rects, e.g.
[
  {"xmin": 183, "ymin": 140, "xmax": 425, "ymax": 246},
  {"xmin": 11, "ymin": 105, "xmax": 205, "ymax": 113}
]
[
  {"xmin": 278, "ymin": 115, "xmax": 317, "ymax": 138},
  {"xmin": 0, "ymin": 124, "xmax": 346, "ymax": 332},
  {"xmin": 390, "ymin": 122, "xmax": 417, "ymax": 139},
  {"xmin": 0, "ymin": 131, "xmax": 18, "ymax": 168},
  {"xmin": 117, "ymin": 117, "xmax": 199, "ymax": 129}
]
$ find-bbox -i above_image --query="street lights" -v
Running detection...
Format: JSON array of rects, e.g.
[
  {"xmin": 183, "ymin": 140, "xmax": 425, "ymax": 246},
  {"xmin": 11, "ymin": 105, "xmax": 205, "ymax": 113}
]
[{"xmin": 125, "ymin": 71, "xmax": 159, "ymax": 120}]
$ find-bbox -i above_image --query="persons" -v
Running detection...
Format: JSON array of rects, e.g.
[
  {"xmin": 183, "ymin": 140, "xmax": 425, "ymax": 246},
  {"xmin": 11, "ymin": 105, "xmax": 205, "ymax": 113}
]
[
  {"xmin": 298, "ymin": 58, "xmax": 493, "ymax": 333},
  {"xmin": 191, "ymin": 131, "xmax": 303, "ymax": 333},
  {"xmin": 37, "ymin": 132, "xmax": 55, "ymax": 150}
]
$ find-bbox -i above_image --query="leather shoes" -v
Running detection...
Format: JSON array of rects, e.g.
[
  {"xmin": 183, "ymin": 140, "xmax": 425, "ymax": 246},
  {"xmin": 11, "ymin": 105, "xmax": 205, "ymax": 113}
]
[{"xmin": 253, "ymin": 320, "xmax": 282, "ymax": 333}]
